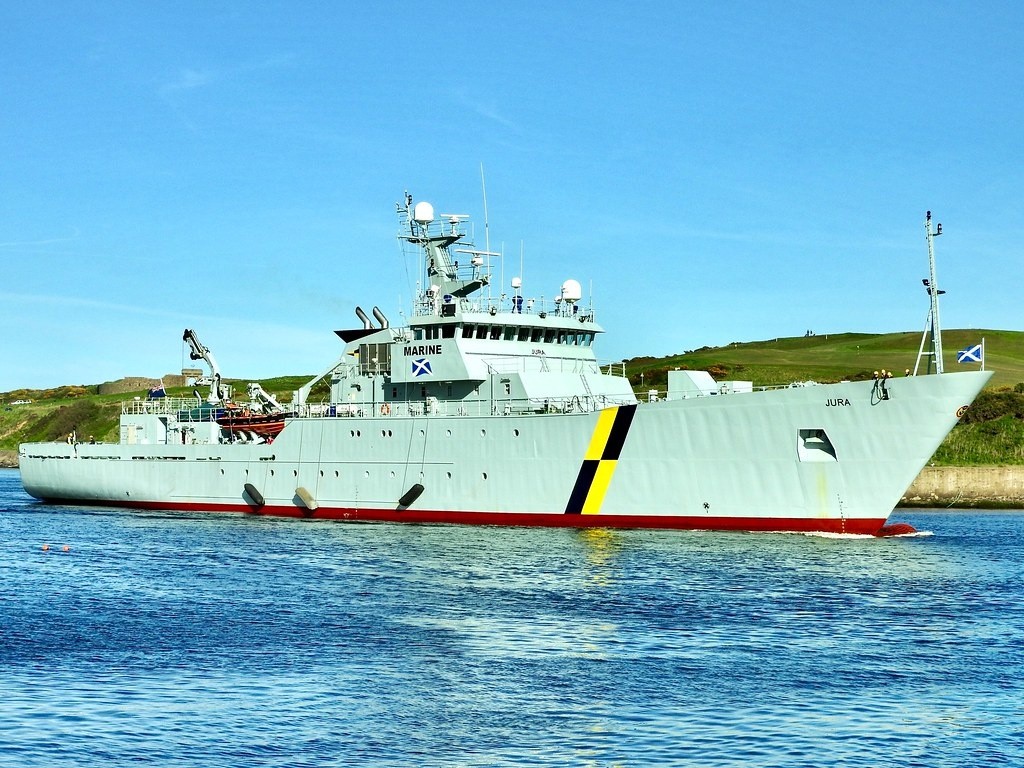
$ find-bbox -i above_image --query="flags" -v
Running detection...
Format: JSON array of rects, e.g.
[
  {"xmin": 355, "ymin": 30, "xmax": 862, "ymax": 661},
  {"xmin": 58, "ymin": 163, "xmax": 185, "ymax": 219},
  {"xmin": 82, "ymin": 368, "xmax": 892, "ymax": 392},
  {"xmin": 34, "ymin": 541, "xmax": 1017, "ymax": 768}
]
[
  {"xmin": 957, "ymin": 343, "xmax": 982, "ymax": 363},
  {"xmin": 147, "ymin": 385, "xmax": 166, "ymax": 398}
]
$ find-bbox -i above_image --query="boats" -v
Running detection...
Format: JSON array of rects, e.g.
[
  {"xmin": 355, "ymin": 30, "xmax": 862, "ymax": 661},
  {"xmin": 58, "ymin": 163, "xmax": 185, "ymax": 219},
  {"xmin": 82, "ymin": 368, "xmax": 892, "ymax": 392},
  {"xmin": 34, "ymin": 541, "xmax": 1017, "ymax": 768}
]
[{"xmin": 18, "ymin": 159, "xmax": 997, "ymax": 539}]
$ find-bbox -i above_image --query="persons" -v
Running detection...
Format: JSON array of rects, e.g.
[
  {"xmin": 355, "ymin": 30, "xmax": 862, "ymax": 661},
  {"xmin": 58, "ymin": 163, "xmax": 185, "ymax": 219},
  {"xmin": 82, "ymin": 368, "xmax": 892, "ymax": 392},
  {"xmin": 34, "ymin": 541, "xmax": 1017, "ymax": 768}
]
[
  {"xmin": 872, "ymin": 371, "xmax": 880, "ymax": 380},
  {"xmin": 89, "ymin": 435, "xmax": 95, "ymax": 444},
  {"xmin": 885, "ymin": 372, "xmax": 893, "ymax": 378},
  {"xmin": 65, "ymin": 433, "xmax": 73, "ymax": 444},
  {"xmin": 881, "ymin": 369, "xmax": 889, "ymax": 378},
  {"xmin": 905, "ymin": 369, "xmax": 913, "ymax": 377},
  {"xmin": 543, "ymin": 400, "xmax": 549, "ymax": 413}
]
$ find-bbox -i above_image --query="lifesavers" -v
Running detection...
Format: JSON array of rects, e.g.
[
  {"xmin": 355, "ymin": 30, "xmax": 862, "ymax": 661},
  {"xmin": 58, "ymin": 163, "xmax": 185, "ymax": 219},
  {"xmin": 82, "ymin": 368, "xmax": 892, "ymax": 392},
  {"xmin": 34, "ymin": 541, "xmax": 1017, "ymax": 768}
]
[{"xmin": 380, "ymin": 404, "xmax": 390, "ymax": 415}]
